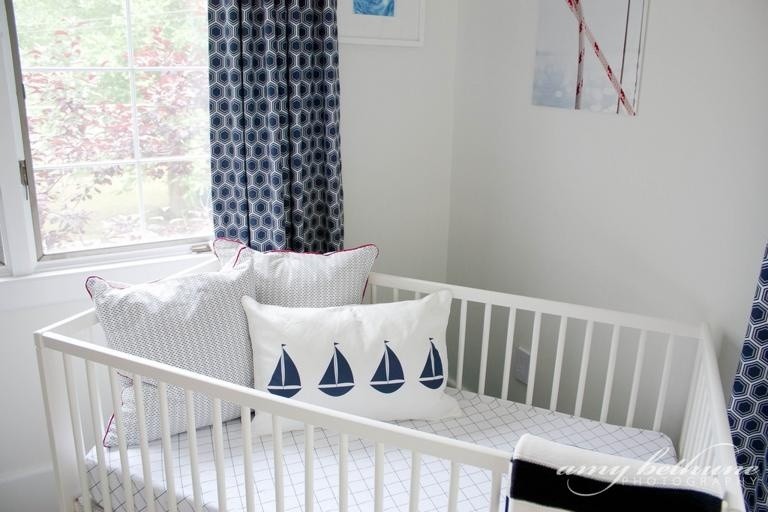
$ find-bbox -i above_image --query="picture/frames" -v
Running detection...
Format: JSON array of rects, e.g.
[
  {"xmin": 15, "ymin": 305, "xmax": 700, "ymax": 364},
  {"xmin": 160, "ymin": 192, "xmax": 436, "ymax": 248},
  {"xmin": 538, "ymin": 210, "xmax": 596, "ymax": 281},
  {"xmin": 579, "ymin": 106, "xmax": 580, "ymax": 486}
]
[{"xmin": 336, "ymin": 1, "xmax": 426, "ymax": 48}]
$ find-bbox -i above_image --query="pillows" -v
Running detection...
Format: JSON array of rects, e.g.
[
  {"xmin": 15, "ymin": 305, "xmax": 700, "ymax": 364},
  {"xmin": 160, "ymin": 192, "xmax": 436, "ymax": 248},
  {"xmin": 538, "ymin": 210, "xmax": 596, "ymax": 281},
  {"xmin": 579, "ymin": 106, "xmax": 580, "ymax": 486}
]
[
  {"xmin": 209, "ymin": 237, "xmax": 379, "ymax": 308},
  {"xmin": 238, "ymin": 289, "xmax": 455, "ymax": 439},
  {"xmin": 85, "ymin": 247, "xmax": 254, "ymax": 448}
]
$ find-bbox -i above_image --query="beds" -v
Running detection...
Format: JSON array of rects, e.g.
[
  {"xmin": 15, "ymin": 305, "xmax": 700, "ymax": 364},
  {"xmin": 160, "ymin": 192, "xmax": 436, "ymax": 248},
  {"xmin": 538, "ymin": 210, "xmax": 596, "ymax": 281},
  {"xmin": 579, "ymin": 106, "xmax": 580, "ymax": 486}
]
[{"xmin": 32, "ymin": 252, "xmax": 746, "ymax": 511}]
